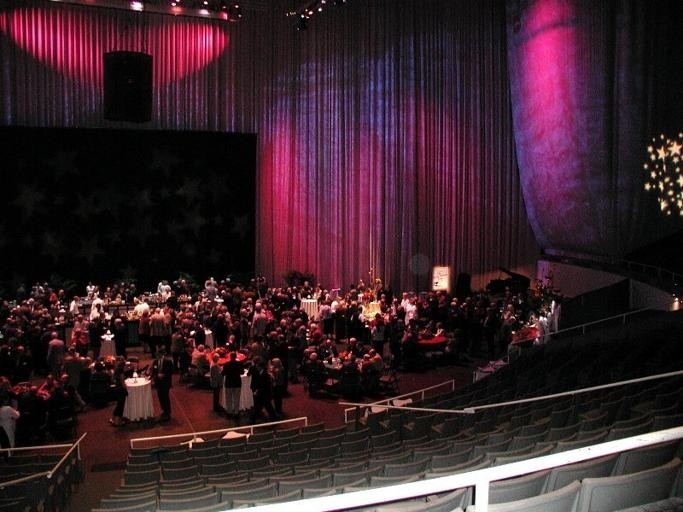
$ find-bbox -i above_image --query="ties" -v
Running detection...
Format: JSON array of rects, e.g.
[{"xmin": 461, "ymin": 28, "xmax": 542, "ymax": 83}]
[{"xmin": 159, "ymin": 359, "xmax": 161, "ymax": 369}]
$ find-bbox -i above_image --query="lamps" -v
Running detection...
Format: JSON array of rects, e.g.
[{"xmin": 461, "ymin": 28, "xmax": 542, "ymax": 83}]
[{"xmin": 133, "ymin": 372, "xmax": 138, "ymax": 383}]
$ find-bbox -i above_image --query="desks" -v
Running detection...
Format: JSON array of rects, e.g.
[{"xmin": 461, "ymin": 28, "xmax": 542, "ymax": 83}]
[
  {"xmin": 219, "ymin": 369, "xmax": 254, "ymax": 410},
  {"xmin": 473, "ymin": 360, "xmax": 508, "ymax": 381},
  {"xmin": 123, "ymin": 377, "xmax": 152, "ymax": 420},
  {"xmin": 189, "ymin": 330, "xmax": 211, "ymax": 347}
]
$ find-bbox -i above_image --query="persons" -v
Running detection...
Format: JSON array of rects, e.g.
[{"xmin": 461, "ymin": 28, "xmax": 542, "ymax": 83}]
[
  {"xmin": 344, "ymin": 338, "xmax": 357, "ymax": 358},
  {"xmin": 354, "ymin": 342, "xmax": 368, "ymax": 359},
  {"xmin": 91, "ymin": 360, "xmax": 109, "ymax": 381},
  {"xmin": 148, "ymin": 348, "xmax": 176, "ymax": 424},
  {"xmin": 89, "ymin": 317, "xmax": 106, "ymax": 360},
  {"xmin": 342, "ymin": 354, "xmax": 355, "ymax": 372},
  {"xmin": 369, "ymin": 349, "xmax": 385, "ymax": 378},
  {"xmin": 109, "ymin": 309, "xmax": 121, "ymax": 334},
  {"xmin": 65, "ymin": 341, "xmax": 85, "ymax": 357},
  {"xmin": 113, "ymin": 319, "xmax": 128, "ymax": 360},
  {"xmin": 139, "ymin": 309, "xmax": 150, "ymax": 352},
  {"xmin": 109, "ymin": 360, "xmax": 128, "ymax": 426},
  {"xmin": 319, "ymin": 340, "xmax": 339, "ymax": 359},
  {"xmin": 191, "ymin": 344, "xmax": 208, "ymax": 378},
  {"xmin": 221, "ymin": 351, "xmax": 245, "ymax": 418},
  {"xmin": 171, "ymin": 273, "xmax": 311, "ymax": 358},
  {"xmin": 58, "ymin": 374, "xmax": 74, "ymax": 402},
  {"xmin": 20, "ymin": 387, "xmax": 46, "ymax": 437},
  {"xmin": 48, "ymin": 384, "xmax": 68, "ymax": 423},
  {"xmin": 71, "ymin": 314, "xmax": 89, "ymax": 343},
  {"xmin": 80, "ymin": 357, "xmax": 91, "ymax": 375},
  {"xmin": 163, "ymin": 307, "xmax": 172, "ymax": 356},
  {"xmin": 249, "ymin": 363, "xmax": 276, "ymax": 420},
  {"xmin": 303, "ymin": 348, "xmax": 313, "ymax": 365},
  {"xmin": 210, "ymin": 353, "xmax": 223, "ymax": 413},
  {"xmin": 65, "ymin": 347, "xmax": 86, "ymax": 413},
  {"xmin": 0, "ymin": 391, "xmax": 20, "ymax": 448},
  {"xmin": 71, "ymin": 327, "xmax": 88, "ymax": 342},
  {"xmin": 270, "ymin": 357, "xmax": 287, "ymax": 417},
  {"xmin": 1, "ymin": 281, "xmax": 71, "ymax": 379},
  {"xmin": 247, "ymin": 356, "xmax": 264, "ymax": 407},
  {"xmin": 98, "ymin": 312, "xmax": 108, "ymax": 335},
  {"xmin": 70, "ymin": 295, "xmax": 82, "ymax": 324},
  {"xmin": 308, "ymin": 280, "xmax": 569, "ymax": 343},
  {"xmin": 359, "ymin": 354, "xmax": 376, "ymax": 380},
  {"xmin": 38, "ymin": 373, "xmax": 56, "ymax": 398},
  {"xmin": 85, "ymin": 279, "xmax": 170, "ymax": 322},
  {"xmin": 306, "ymin": 352, "xmax": 328, "ymax": 394},
  {"xmin": 149, "ymin": 307, "xmax": 165, "ymax": 358}
]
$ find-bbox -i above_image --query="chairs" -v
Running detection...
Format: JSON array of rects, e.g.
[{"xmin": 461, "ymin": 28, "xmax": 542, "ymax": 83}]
[
  {"xmin": 94, "ymin": 311, "xmax": 681, "ymax": 512},
  {"xmin": 379, "ymin": 372, "xmax": 400, "ymax": 395},
  {"xmin": 0, "ymin": 453, "xmax": 85, "ymax": 511}
]
